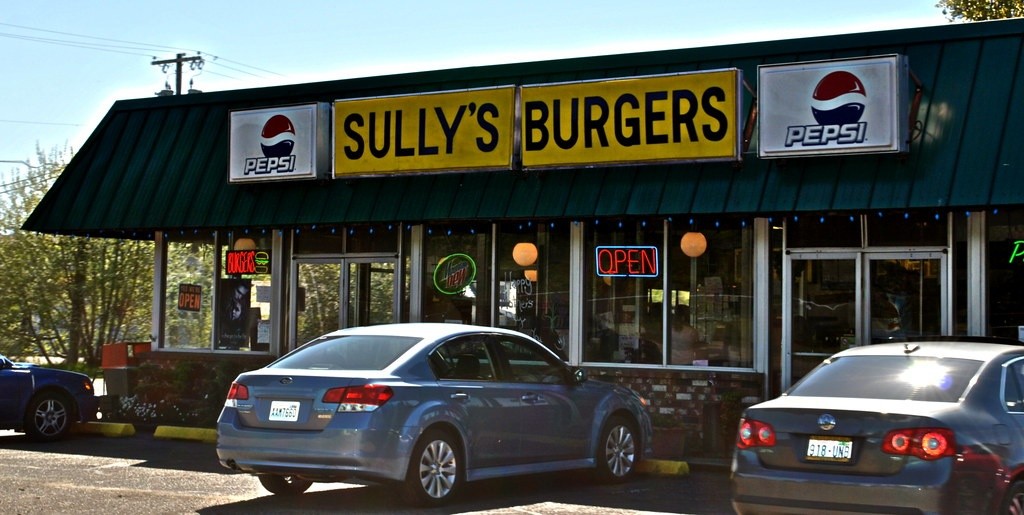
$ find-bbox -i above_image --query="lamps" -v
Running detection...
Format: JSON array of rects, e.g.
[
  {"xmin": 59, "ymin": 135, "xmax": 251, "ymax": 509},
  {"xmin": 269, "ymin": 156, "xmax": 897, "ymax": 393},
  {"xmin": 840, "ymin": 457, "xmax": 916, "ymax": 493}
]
[
  {"xmin": 235, "ymin": 233, "xmax": 256, "ymax": 250},
  {"xmin": 680, "ymin": 225, "xmax": 707, "ymax": 258},
  {"xmin": 512, "ymin": 234, "xmax": 538, "ymax": 266},
  {"xmin": 525, "ymin": 270, "xmax": 537, "ymax": 282}
]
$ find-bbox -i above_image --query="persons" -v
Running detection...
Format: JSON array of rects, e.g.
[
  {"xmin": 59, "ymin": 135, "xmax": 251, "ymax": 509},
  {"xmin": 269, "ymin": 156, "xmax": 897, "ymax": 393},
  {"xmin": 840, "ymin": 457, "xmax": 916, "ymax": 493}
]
[{"xmin": 670, "ymin": 304, "xmax": 697, "ymax": 366}]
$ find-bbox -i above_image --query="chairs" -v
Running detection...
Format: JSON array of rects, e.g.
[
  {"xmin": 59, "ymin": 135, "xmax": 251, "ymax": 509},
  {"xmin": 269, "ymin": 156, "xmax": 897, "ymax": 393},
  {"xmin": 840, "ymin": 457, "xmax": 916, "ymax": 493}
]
[{"xmin": 454, "ymin": 354, "xmax": 481, "ymax": 379}]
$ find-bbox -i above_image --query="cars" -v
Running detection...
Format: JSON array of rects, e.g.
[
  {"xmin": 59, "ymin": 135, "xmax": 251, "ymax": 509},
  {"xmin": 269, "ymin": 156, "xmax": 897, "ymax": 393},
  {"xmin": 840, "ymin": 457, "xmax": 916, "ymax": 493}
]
[
  {"xmin": 1, "ymin": 355, "xmax": 95, "ymax": 444},
  {"xmin": 216, "ymin": 323, "xmax": 651, "ymax": 511},
  {"xmin": 730, "ymin": 338, "xmax": 1024, "ymax": 515}
]
